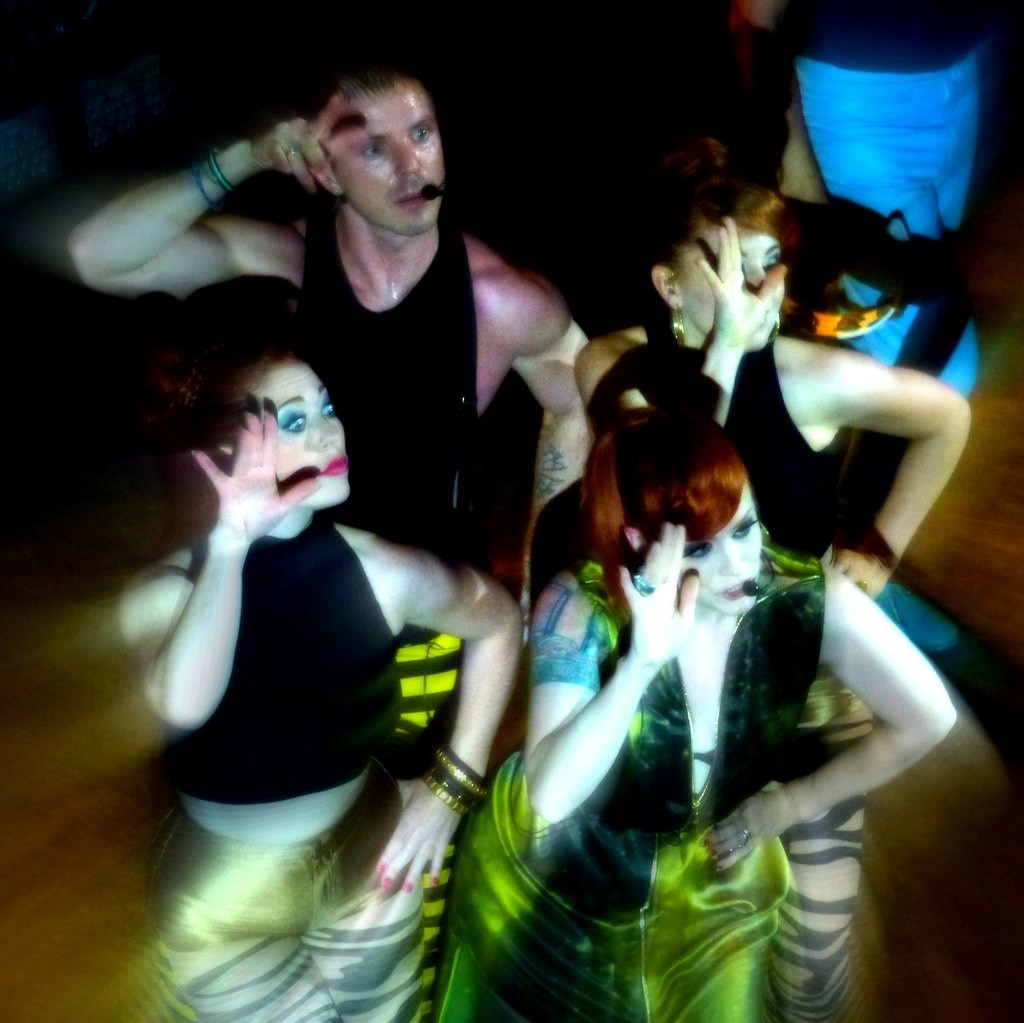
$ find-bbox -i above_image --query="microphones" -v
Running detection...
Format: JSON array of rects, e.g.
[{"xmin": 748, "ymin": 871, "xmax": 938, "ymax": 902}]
[
  {"xmin": 743, "ymin": 550, "xmax": 775, "ymax": 596},
  {"xmin": 421, "ymin": 184, "xmax": 447, "ymax": 201}
]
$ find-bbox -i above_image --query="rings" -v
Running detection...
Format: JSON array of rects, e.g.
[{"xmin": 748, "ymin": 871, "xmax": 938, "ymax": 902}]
[
  {"xmin": 855, "ymin": 580, "xmax": 867, "ymax": 591},
  {"xmin": 633, "ymin": 567, "xmax": 655, "ymax": 596},
  {"xmin": 742, "ymin": 830, "xmax": 751, "ymax": 841},
  {"xmin": 286, "ymin": 146, "xmax": 299, "ymax": 157}
]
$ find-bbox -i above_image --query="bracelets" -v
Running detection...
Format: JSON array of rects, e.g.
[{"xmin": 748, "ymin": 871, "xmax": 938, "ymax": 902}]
[
  {"xmin": 424, "ymin": 744, "xmax": 488, "ymax": 816},
  {"xmin": 191, "ymin": 161, "xmax": 223, "ymax": 212},
  {"xmin": 207, "ymin": 148, "xmax": 233, "ymax": 192}
]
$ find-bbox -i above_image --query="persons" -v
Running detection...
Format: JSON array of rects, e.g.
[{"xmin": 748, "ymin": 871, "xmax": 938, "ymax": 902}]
[{"xmin": 72, "ymin": 0, "xmax": 1024, "ymax": 1023}]
